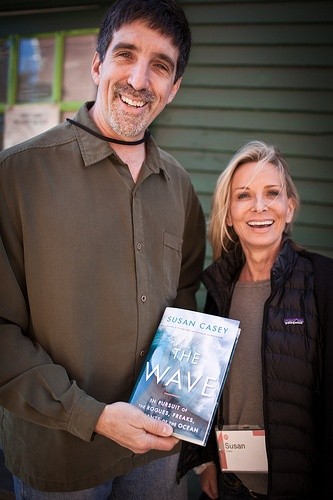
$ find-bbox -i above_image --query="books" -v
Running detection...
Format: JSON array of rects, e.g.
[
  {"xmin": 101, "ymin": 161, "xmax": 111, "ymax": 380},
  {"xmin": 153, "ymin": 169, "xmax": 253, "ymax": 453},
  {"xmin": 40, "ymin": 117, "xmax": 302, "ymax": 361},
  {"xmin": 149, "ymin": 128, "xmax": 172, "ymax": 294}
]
[{"xmin": 128, "ymin": 305, "xmax": 241, "ymax": 449}]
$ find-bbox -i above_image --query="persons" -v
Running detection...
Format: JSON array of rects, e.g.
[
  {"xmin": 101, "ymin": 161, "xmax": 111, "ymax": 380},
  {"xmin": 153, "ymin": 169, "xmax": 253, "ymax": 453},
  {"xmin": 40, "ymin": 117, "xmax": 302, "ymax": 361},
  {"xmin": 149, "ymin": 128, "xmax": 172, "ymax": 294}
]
[
  {"xmin": 0, "ymin": 1, "xmax": 208, "ymax": 498},
  {"xmin": 176, "ymin": 138, "xmax": 333, "ymax": 500}
]
